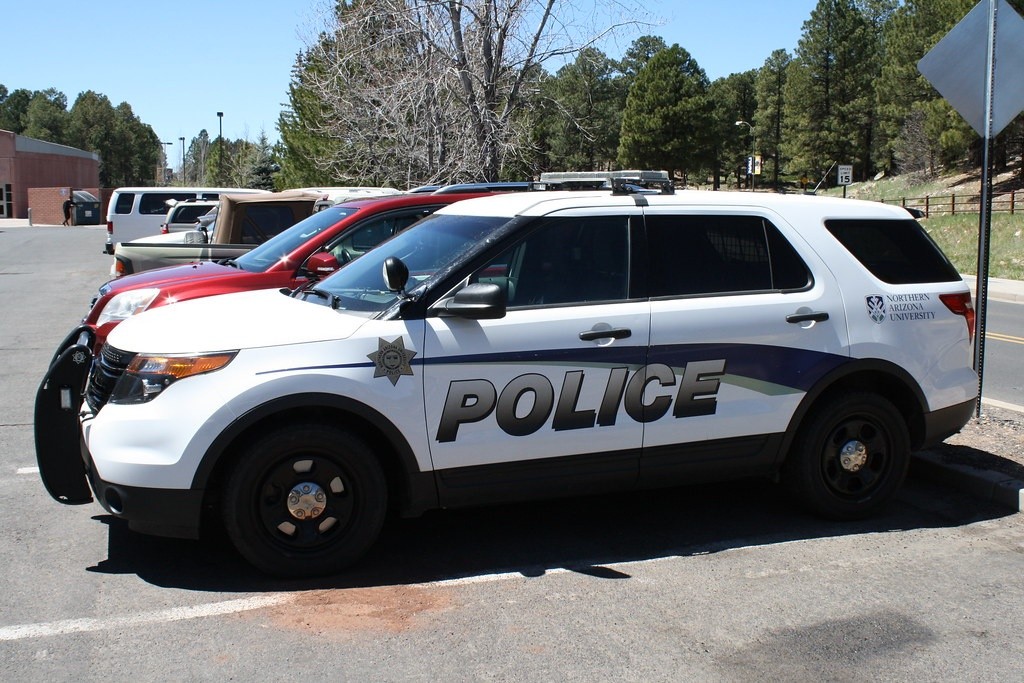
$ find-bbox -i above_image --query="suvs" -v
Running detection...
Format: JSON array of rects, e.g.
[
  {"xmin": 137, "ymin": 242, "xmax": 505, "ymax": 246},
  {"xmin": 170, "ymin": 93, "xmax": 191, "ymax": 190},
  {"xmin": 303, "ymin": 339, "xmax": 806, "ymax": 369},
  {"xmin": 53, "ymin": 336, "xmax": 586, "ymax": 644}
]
[
  {"xmin": 160, "ymin": 198, "xmax": 220, "ymax": 234},
  {"xmin": 75, "ymin": 180, "xmax": 729, "ymax": 372}
]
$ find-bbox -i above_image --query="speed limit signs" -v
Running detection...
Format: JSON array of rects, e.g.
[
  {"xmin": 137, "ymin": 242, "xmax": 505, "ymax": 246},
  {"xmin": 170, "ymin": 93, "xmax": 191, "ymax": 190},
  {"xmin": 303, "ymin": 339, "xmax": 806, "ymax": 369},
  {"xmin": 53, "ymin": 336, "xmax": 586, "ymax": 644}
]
[{"xmin": 838, "ymin": 165, "xmax": 852, "ymax": 185}]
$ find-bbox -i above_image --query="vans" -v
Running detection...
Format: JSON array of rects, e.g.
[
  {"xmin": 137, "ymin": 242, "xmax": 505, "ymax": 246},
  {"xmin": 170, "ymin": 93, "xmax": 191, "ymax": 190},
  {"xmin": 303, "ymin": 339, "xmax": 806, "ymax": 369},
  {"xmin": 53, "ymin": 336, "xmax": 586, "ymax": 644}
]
[{"xmin": 103, "ymin": 186, "xmax": 274, "ymax": 256}]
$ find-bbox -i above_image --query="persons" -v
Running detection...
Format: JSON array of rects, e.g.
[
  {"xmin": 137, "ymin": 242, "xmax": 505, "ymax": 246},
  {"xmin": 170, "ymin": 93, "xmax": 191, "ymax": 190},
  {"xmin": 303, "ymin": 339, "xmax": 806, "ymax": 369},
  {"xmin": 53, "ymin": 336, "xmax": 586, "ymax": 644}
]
[{"xmin": 62, "ymin": 196, "xmax": 77, "ymax": 227}]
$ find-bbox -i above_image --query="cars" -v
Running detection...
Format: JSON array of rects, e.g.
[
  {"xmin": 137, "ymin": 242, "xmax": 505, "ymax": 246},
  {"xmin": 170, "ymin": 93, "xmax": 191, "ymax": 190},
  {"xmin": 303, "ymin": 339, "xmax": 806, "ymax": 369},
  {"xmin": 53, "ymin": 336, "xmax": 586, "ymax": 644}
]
[{"xmin": 76, "ymin": 169, "xmax": 979, "ymax": 588}]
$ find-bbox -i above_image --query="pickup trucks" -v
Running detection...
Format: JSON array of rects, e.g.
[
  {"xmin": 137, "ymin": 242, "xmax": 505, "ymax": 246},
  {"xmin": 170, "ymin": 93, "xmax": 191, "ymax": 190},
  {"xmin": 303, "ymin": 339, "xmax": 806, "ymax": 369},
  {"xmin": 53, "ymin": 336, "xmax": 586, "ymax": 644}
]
[{"xmin": 110, "ymin": 196, "xmax": 425, "ymax": 282}]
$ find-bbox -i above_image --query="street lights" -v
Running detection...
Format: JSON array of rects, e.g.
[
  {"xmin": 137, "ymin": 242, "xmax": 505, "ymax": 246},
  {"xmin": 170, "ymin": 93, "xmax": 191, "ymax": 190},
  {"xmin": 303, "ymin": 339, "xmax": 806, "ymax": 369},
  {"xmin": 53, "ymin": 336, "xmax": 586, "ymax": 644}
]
[
  {"xmin": 217, "ymin": 112, "xmax": 224, "ymax": 166},
  {"xmin": 179, "ymin": 137, "xmax": 186, "ymax": 186},
  {"xmin": 160, "ymin": 142, "xmax": 173, "ymax": 184},
  {"xmin": 736, "ymin": 121, "xmax": 755, "ymax": 192}
]
